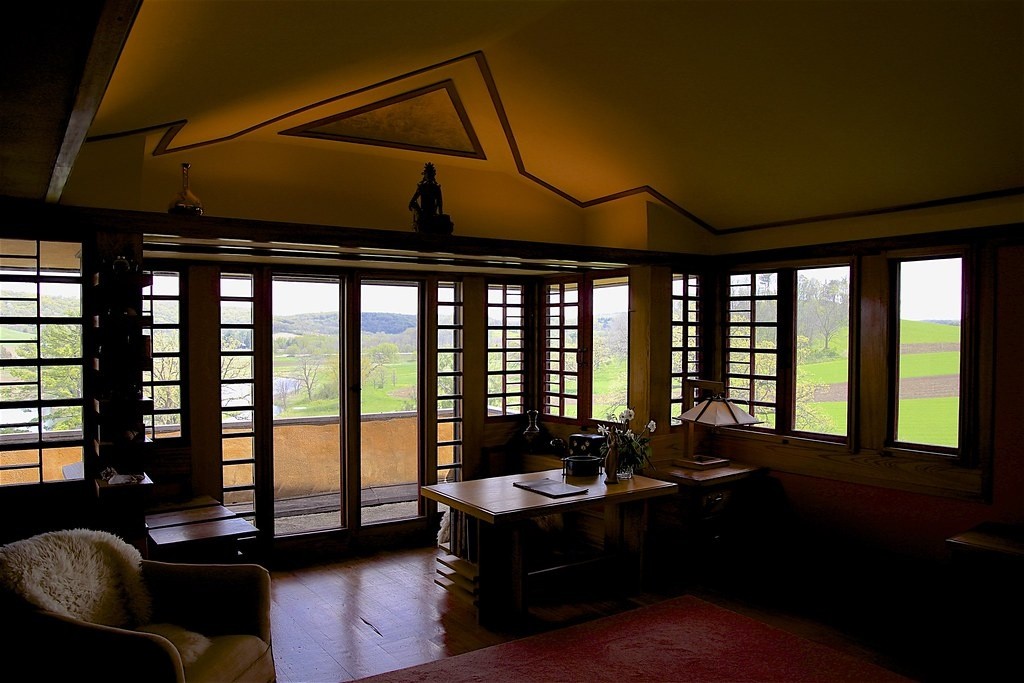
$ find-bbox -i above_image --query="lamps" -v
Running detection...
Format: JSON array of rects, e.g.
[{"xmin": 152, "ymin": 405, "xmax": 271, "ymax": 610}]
[
  {"xmin": 168, "ymin": 162, "xmax": 202, "ymax": 218},
  {"xmin": 671, "ymin": 378, "xmax": 764, "ymax": 471}
]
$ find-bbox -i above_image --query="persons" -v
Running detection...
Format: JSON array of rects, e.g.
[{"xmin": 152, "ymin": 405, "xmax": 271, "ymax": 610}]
[{"xmin": 604, "ymin": 425, "xmax": 620, "ymax": 474}]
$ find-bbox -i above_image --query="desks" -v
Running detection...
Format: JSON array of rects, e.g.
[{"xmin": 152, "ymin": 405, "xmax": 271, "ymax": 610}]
[
  {"xmin": 420, "ymin": 452, "xmax": 680, "ymax": 630},
  {"xmin": 652, "ymin": 461, "xmax": 768, "ymax": 588}
]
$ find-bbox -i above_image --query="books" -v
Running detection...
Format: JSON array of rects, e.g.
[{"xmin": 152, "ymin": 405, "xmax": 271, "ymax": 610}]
[{"xmin": 513, "ymin": 478, "xmax": 589, "ymax": 499}]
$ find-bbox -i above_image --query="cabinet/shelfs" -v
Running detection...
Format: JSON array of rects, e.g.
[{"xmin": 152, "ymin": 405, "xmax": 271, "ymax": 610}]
[{"xmin": 92, "ymin": 268, "xmax": 157, "ymax": 500}]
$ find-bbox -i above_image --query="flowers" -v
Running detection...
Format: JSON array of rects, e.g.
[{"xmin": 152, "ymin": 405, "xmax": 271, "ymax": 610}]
[{"xmin": 597, "ymin": 409, "xmax": 656, "ymax": 476}]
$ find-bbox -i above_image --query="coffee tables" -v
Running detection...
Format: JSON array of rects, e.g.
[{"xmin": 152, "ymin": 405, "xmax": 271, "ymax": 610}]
[{"xmin": 142, "ymin": 494, "xmax": 260, "ymax": 560}]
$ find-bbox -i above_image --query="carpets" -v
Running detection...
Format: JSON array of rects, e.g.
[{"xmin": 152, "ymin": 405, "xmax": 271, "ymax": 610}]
[{"xmin": 339, "ymin": 595, "xmax": 911, "ymax": 683}]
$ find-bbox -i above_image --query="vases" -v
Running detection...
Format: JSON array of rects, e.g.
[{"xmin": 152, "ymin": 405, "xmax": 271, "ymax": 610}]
[{"xmin": 617, "ymin": 463, "xmax": 633, "ymax": 479}]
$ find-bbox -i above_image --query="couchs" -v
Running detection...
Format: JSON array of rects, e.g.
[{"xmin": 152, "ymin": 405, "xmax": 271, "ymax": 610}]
[{"xmin": 0, "ymin": 528, "xmax": 277, "ymax": 683}]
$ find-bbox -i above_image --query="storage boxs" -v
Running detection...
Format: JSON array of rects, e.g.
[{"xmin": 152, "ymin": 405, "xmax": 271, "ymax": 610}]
[{"xmin": 568, "ymin": 433, "xmax": 605, "ymax": 456}]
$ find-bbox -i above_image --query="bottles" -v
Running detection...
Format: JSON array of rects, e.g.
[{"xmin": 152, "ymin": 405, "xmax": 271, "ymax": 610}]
[
  {"xmin": 169, "ymin": 163, "xmax": 203, "ymax": 216},
  {"xmin": 523, "ymin": 409, "xmax": 540, "ymax": 455}
]
